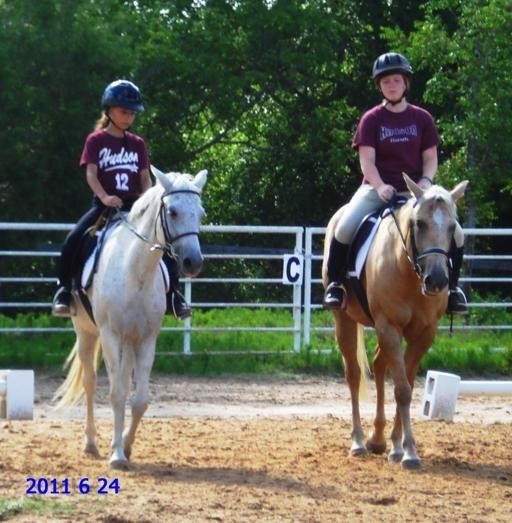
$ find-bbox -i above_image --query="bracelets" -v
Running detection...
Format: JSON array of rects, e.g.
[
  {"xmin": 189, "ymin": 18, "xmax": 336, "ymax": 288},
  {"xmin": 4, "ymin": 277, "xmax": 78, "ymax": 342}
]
[{"xmin": 421, "ymin": 174, "xmax": 436, "ymax": 185}]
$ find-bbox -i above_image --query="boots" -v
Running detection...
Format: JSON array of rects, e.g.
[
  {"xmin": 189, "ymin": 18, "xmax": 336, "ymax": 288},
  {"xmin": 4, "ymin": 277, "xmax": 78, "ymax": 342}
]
[
  {"xmin": 170, "ymin": 265, "xmax": 191, "ymax": 315},
  {"xmin": 450, "ymin": 245, "xmax": 467, "ymax": 314},
  {"xmin": 54, "ymin": 245, "xmax": 73, "ymax": 310},
  {"xmin": 324, "ymin": 237, "xmax": 350, "ymax": 306}
]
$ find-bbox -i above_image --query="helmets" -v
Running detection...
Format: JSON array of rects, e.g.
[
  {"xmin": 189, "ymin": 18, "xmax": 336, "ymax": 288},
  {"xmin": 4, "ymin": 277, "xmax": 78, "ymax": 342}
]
[
  {"xmin": 100, "ymin": 78, "xmax": 145, "ymax": 112},
  {"xmin": 372, "ymin": 51, "xmax": 413, "ymax": 77}
]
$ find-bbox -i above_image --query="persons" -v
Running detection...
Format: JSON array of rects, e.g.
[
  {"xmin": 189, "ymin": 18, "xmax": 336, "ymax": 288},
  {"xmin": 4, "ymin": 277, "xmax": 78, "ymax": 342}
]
[
  {"xmin": 49, "ymin": 78, "xmax": 194, "ymax": 320},
  {"xmin": 322, "ymin": 50, "xmax": 470, "ymax": 314}
]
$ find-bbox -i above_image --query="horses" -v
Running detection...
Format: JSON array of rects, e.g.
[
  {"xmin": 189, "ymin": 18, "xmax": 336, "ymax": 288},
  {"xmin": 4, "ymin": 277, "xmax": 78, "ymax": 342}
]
[
  {"xmin": 50, "ymin": 163, "xmax": 209, "ymax": 474},
  {"xmin": 321, "ymin": 169, "xmax": 471, "ymax": 470}
]
